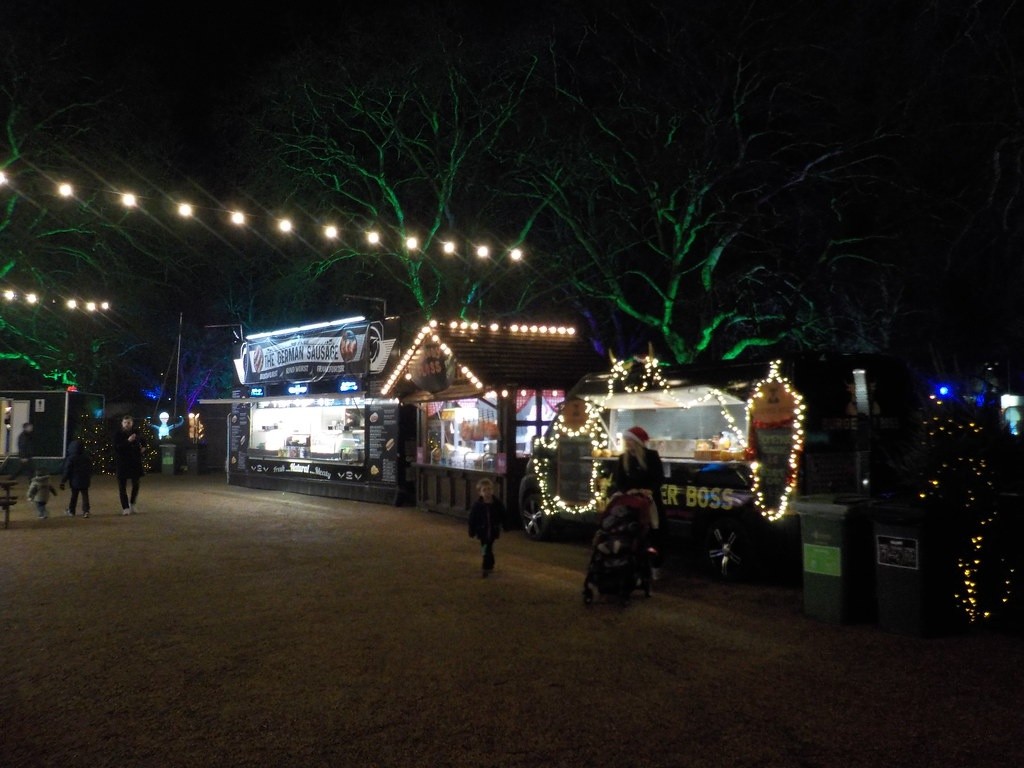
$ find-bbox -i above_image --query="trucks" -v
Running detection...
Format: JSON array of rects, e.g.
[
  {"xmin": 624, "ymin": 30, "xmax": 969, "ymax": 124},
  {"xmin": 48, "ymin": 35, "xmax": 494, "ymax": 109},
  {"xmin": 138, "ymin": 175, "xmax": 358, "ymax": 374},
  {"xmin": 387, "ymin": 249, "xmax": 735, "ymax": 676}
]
[{"xmin": 518, "ymin": 374, "xmax": 795, "ymax": 583}]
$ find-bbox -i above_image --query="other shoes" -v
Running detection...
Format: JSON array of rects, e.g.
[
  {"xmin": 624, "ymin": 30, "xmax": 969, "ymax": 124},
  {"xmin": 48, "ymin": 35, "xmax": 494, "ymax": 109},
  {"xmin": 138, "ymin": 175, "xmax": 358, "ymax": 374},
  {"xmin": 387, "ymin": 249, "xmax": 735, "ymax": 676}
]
[
  {"xmin": 483, "ymin": 570, "xmax": 489, "ymax": 578},
  {"xmin": 123, "ymin": 510, "xmax": 129, "ymax": 516},
  {"xmin": 65, "ymin": 508, "xmax": 73, "ymax": 517},
  {"xmin": 39, "ymin": 512, "xmax": 47, "ymax": 518},
  {"xmin": 130, "ymin": 506, "xmax": 140, "ymax": 514},
  {"xmin": 488, "ymin": 559, "xmax": 494, "ymax": 569},
  {"xmin": 83, "ymin": 512, "xmax": 89, "ymax": 518}
]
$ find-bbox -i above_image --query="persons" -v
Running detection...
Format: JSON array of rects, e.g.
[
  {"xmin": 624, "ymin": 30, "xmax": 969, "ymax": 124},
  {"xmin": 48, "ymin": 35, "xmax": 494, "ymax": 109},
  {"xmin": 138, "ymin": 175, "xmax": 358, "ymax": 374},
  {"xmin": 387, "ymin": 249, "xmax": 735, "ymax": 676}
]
[
  {"xmin": 26, "ymin": 468, "xmax": 58, "ymax": 519},
  {"xmin": 606, "ymin": 426, "xmax": 670, "ymax": 600},
  {"xmin": 467, "ymin": 478, "xmax": 511, "ymax": 579},
  {"xmin": 114, "ymin": 415, "xmax": 148, "ymax": 515},
  {"xmin": 17, "ymin": 422, "xmax": 36, "ymax": 483},
  {"xmin": 59, "ymin": 440, "xmax": 91, "ymax": 518}
]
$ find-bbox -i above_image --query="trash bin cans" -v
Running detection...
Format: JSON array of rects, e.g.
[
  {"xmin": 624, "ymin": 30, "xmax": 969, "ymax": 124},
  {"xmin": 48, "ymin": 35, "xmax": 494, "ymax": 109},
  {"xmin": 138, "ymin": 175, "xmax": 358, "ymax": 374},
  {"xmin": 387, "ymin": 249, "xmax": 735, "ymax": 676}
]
[
  {"xmin": 794, "ymin": 494, "xmax": 868, "ymax": 624},
  {"xmin": 160, "ymin": 443, "xmax": 177, "ymax": 474},
  {"xmin": 185, "ymin": 449, "xmax": 198, "ymax": 475},
  {"xmin": 869, "ymin": 502, "xmax": 943, "ymax": 634}
]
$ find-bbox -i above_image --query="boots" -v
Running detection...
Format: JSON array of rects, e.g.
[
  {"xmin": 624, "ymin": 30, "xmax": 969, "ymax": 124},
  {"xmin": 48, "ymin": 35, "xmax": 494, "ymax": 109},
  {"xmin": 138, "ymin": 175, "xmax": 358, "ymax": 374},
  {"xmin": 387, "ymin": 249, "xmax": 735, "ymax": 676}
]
[{"xmin": 643, "ymin": 576, "xmax": 653, "ymax": 600}]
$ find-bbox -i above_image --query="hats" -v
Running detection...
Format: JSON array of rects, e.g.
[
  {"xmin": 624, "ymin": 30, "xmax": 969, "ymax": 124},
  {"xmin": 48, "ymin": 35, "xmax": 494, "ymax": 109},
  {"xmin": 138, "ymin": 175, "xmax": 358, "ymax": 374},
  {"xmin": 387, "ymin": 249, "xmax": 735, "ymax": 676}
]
[{"xmin": 627, "ymin": 427, "xmax": 649, "ymax": 447}]
